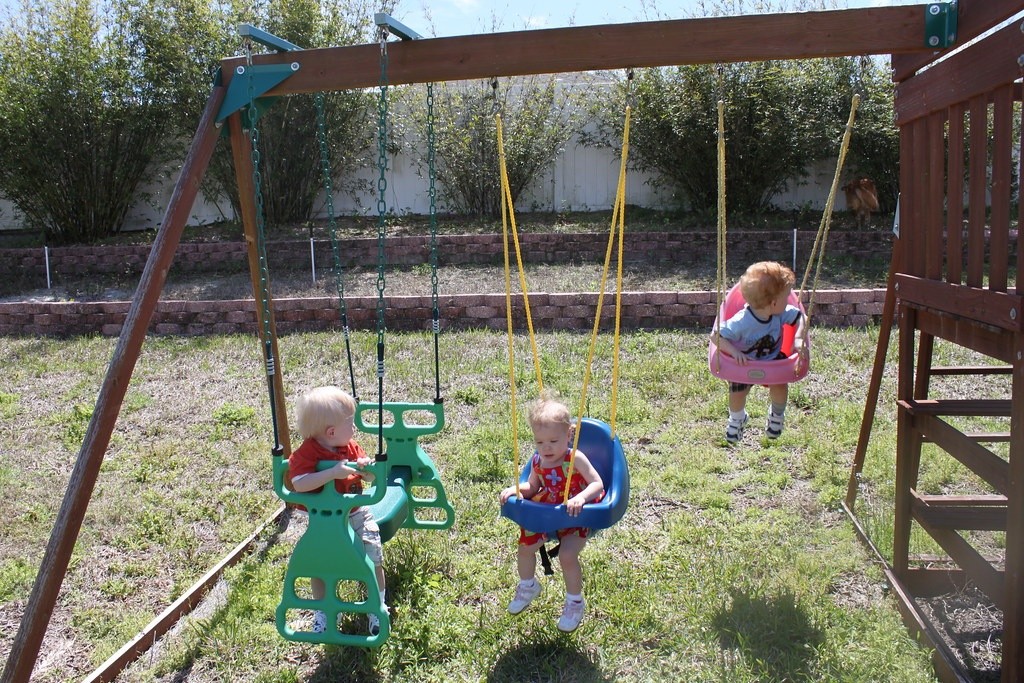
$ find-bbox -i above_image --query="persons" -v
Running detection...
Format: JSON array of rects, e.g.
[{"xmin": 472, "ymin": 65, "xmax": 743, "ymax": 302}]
[
  {"xmin": 288, "ymin": 385, "xmax": 391, "ymax": 635},
  {"xmin": 500, "ymin": 400, "xmax": 605, "ymax": 633},
  {"xmin": 711, "ymin": 262, "xmax": 805, "ymax": 445}
]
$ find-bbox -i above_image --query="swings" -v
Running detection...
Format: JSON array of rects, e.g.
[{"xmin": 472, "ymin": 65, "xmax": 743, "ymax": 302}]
[
  {"xmin": 706, "ymin": 57, "xmax": 868, "ymax": 385},
  {"xmin": 242, "ymin": 43, "xmax": 459, "ymax": 649},
  {"xmin": 497, "ymin": 67, "xmax": 628, "ymax": 535}
]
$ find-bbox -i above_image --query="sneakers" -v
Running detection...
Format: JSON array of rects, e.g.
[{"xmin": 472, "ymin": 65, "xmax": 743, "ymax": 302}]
[
  {"xmin": 765, "ymin": 407, "xmax": 785, "ymax": 439},
  {"xmin": 556, "ymin": 597, "xmax": 584, "ymax": 633},
  {"xmin": 313, "ymin": 610, "xmax": 327, "ymax": 633},
  {"xmin": 726, "ymin": 412, "xmax": 749, "ymax": 444},
  {"xmin": 507, "ymin": 578, "xmax": 542, "ymax": 615},
  {"xmin": 366, "ymin": 602, "xmax": 391, "ymax": 636}
]
